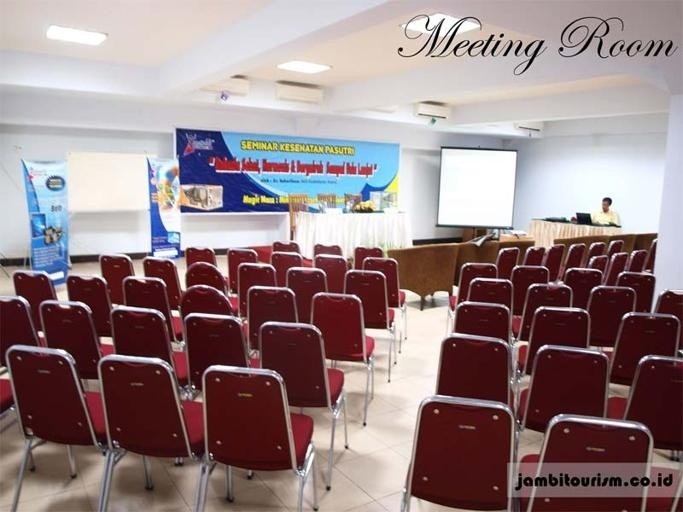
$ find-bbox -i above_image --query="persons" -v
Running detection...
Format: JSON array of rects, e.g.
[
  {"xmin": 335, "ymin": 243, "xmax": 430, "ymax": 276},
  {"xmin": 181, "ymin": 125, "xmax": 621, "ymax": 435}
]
[{"xmin": 592, "ymin": 197, "xmax": 619, "ymax": 225}]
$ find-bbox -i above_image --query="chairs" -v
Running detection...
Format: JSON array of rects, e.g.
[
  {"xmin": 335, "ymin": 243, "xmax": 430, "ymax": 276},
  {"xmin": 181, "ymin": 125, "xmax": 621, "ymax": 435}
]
[
  {"xmin": 344, "ymin": 193, "xmax": 363, "ymax": 213},
  {"xmin": 0, "ymin": 239, "xmax": 405, "ymax": 512},
  {"xmin": 401, "ymin": 232, "xmax": 683, "ymax": 512},
  {"xmin": 317, "ymin": 193, "xmax": 337, "ymax": 213},
  {"xmin": 288, "ymin": 193, "xmax": 309, "ymax": 230}
]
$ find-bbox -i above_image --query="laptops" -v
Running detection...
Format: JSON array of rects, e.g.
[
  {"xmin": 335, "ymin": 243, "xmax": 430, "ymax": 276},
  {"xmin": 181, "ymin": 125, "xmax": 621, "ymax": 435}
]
[{"xmin": 577, "ymin": 213, "xmax": 599, "ymax": 225}]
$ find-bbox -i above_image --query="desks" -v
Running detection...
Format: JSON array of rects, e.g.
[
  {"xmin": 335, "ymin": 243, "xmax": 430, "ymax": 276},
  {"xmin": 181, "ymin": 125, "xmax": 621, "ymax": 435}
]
[
  {"xmin": 529, "ymin": 219, "xmax": 622, "ymax": 254},
  {"xmin": 179, "ymin": 211, "xmax": 291, "ymax": 252},
  {"xmin": 294, "ymin": 211, "xmax": 417, "ymax": 267}
]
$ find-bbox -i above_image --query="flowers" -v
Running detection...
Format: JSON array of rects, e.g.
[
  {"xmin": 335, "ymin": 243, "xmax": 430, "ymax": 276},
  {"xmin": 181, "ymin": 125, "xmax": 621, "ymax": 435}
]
[{"xmin": 353, "ymin": 200, "xmax": 375, "ymax": 212}]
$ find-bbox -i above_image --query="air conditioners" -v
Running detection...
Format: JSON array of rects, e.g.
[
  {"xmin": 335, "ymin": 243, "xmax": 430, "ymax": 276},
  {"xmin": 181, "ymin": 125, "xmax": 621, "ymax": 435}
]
[
  {"xmin": 514, "ymin": 121, "xmax": 544, "ymax": 134},
  {"xmin": 415, "ymin": 101, "xmax": 448, "ymax": 119}
]
